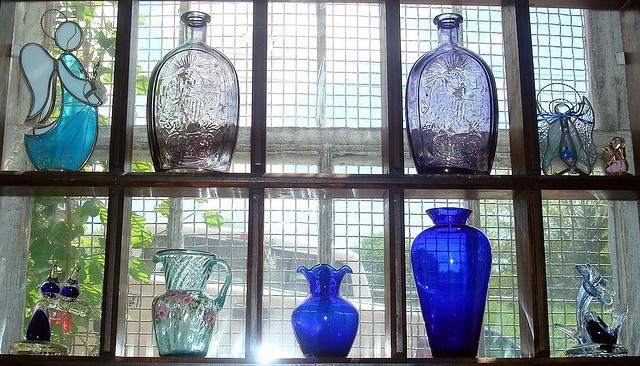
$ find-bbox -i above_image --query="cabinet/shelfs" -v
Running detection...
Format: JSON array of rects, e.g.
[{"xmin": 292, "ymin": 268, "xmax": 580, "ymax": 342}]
[{"xmin": 1, "ymin": 0, "xmax": 639, "ymax": 365}]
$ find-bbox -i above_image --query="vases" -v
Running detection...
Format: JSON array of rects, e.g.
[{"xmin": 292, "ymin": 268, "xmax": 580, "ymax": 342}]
[
  {"xmin": 537, "ymin": 83, "xmax": 598, "ymax": 179},
  {"xmin": 293, "ymin": 263, "xmax": 359, "ymax": 357},
  {"xmin": 26, "ymin": 259, "xmax": 80, "ymax": 339},
  {"xmin": 554, "ymin": 263, "xmax": 629, "ymax": 356},
  {"xmin": 410, "ymin": 207, "xmax": 492, "ymax": 356},
  {"xmin": 20, "ymin": 8, "xmax": 101, "ymax": 168},
  {"xmin": 405, "ymin": 12, "xmax": 499, "ymax": 174},
  {"xmin": 146, "ymin": 11, "xmax": 240, "ymax": 173},
  {"xmin": 152, "ymin": 250, "xmax": 230, "ymax": 355}
]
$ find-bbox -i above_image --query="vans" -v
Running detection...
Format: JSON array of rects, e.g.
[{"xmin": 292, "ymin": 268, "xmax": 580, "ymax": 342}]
[{"xmin": 83, "ymin": 221, "xmax": 426, "ymax": 359}]
